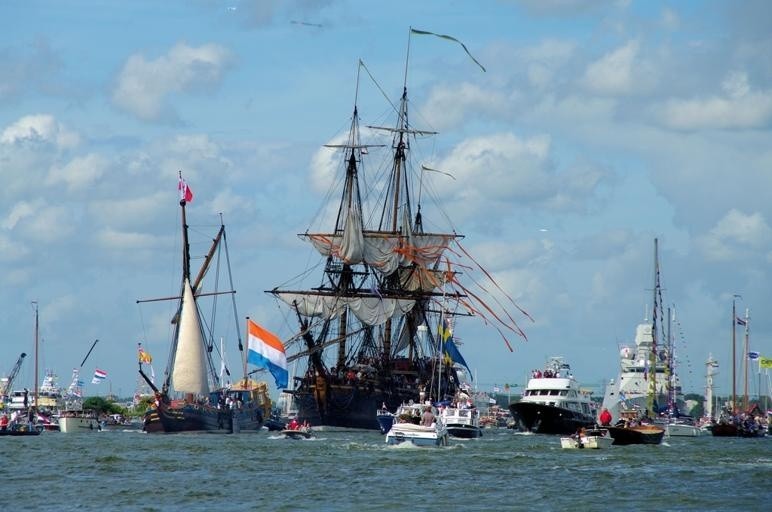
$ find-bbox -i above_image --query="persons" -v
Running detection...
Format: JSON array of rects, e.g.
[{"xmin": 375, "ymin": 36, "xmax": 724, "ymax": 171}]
[
  {"xmin": 284, "ymin": 417, "xmax": 312, "ymax": 434},
  {"xmin": 21, "ymin": 388, "xmax": 28, "ymax": 408},
  {"xmin": 329, "ymin": 347, "xmax": 388, "ymax": 387},
  {"xmin": 395, "ymin": 389, "xmax": 476, "ymax": 428},
  {"xmin": 692, "ymin": 403, "xmax": 767, "ymax": 437},
  {"xmin": 28, "ymin": 405, "xmax": 36, "ymax": 432},
  {"xmin": 0, "ymin": 409, "xmax": 9, "ymax": 430},
  {"xmin": 215, "ymin": 392, "xmax": 246, "ymax": 410},
  {"xmin": 571, "ymin": 403, "xmax": 655, "ymax": 438},
  {"xmin": 529, "ymin": 367, "xmax": 562, "ymax": 380}
]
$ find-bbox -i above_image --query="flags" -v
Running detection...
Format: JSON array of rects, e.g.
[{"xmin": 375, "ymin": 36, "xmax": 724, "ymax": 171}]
[
  {"xmin": 139, "ymin": 346, "xmax": 153, "ymax": 364},
  {"xmin": 246, "ymin": 318, "xmax": 289, "ymax": 389},
  {"xmin": 92, "ymin": 369, "xmax": 107, "ymax": 384},
  {"xmin": 183, "ymin": 184, "xmax": 193, "ymax": 202},
  {"xmin": 748, "ymin": 351, "xmax": 760, "ymax": 360},
  {"xmin": 436, "ymin": 313, "xmax": 474, "ymax": 382},
  {"xmin": 220, "ymin": 337, "xmax": 231, "ymax": 378},
  {"xmin": 178, "ymin": 175, "xmax": 185, "ymax": 191},
  {"xmin": 736, "ymin": 316, "xmax": 746, "ymax": 326},
  {"xmin": 759, "ymin": 356, "xmax": 772, "ymax": 368}
]
